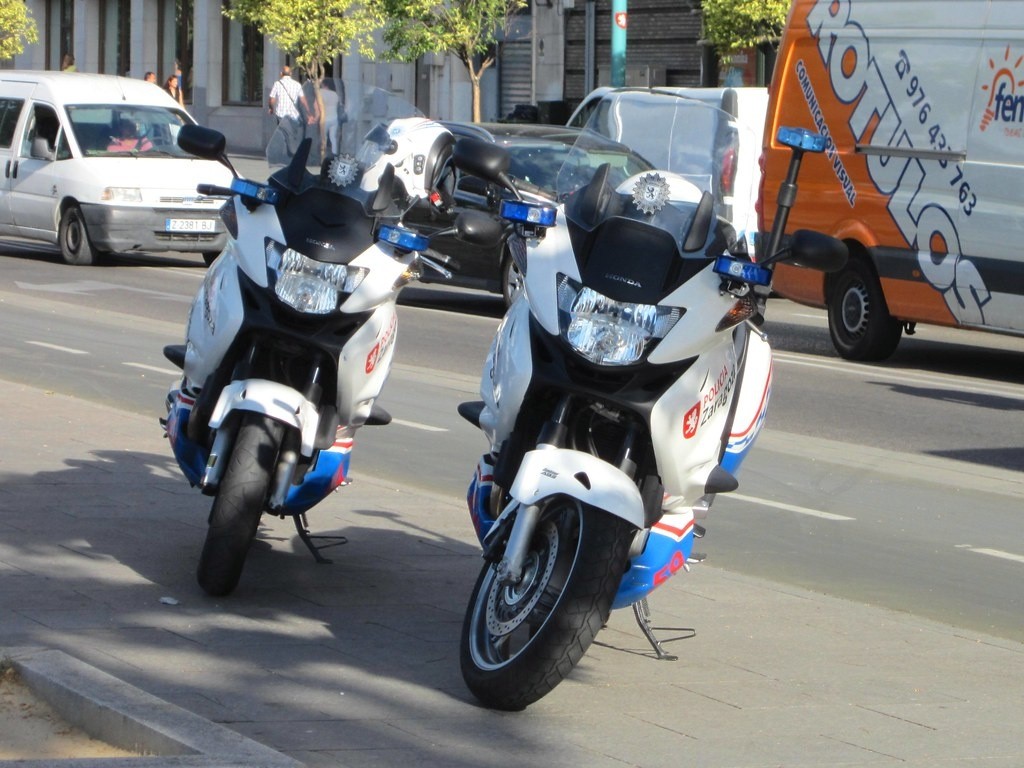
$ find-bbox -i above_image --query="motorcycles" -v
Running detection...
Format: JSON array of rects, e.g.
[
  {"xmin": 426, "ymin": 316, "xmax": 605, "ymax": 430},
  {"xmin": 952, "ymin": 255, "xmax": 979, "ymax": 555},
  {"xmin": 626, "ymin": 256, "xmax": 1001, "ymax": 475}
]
[
  {"xmin": 154, "ymin": 78, "xmax": 504, "ymax": 599},
  {"xmin": 455, "ymin": 87, "xmax": 830, "ymax": 711}
]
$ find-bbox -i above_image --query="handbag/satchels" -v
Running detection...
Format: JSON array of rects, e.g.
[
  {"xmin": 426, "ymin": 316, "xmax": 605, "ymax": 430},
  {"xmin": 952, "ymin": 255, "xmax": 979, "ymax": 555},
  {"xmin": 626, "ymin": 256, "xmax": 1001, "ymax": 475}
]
[{"xmin": 338, "ymin": 109, "xmax": 348, "ymax": 124}]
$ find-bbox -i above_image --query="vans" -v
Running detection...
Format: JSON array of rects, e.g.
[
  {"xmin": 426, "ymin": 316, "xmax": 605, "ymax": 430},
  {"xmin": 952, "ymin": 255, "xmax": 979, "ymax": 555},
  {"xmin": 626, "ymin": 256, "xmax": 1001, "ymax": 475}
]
[
  {"xmin": 0, "ymin": 69, "xmax": 243, "ymax": 269},
  {"xmin": 753, "ymin": 0, "xmax": 1024, "ymax": 365},
  {"xmin": 564, "ymin": 83, "xmax": 775, "ymax": 261}
]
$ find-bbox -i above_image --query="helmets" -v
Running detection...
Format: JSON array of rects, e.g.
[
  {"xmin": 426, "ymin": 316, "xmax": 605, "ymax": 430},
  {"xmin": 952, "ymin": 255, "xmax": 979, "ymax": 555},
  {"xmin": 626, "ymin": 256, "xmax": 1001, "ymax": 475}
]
[
  {"xmin": 616, "ymin": 170, "xmax": 716, "ymax": 258},
  {"xmin": 360, "ymin": 117, "xmax": 455, "ymax": 200}
]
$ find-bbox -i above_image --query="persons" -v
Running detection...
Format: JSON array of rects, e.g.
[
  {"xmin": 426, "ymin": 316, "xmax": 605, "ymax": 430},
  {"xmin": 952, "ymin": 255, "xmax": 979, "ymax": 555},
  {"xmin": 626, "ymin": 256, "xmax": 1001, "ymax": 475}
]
[
  {"xmin": 61, "ymin": 54, "xmax": 80, "ymax": 72},
  {"xmin": 107, "ymin": 120, "xmax": 159, "ymax": 153},
  {"xmin": 163, "ymin": 75, "xmax": 185, "ymax": 124},
  {"xmin": 308, "ymin": 79, "xmax": 342, "ymax": 165},
  {"xmin": 144, "ymin": 72, "xmax": 156, "ymax": 84},
  {"xmin": 268, "ymin": 65, "xmax": 313, "ymax": 165}
]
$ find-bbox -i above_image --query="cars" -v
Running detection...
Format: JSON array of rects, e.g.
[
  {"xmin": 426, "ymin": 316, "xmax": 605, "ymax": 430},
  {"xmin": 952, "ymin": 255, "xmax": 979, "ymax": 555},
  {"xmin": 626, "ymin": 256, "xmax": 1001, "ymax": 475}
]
[{"xmin": 358, "ymin": 120, "xmax": 719, "ymax": 316}]
